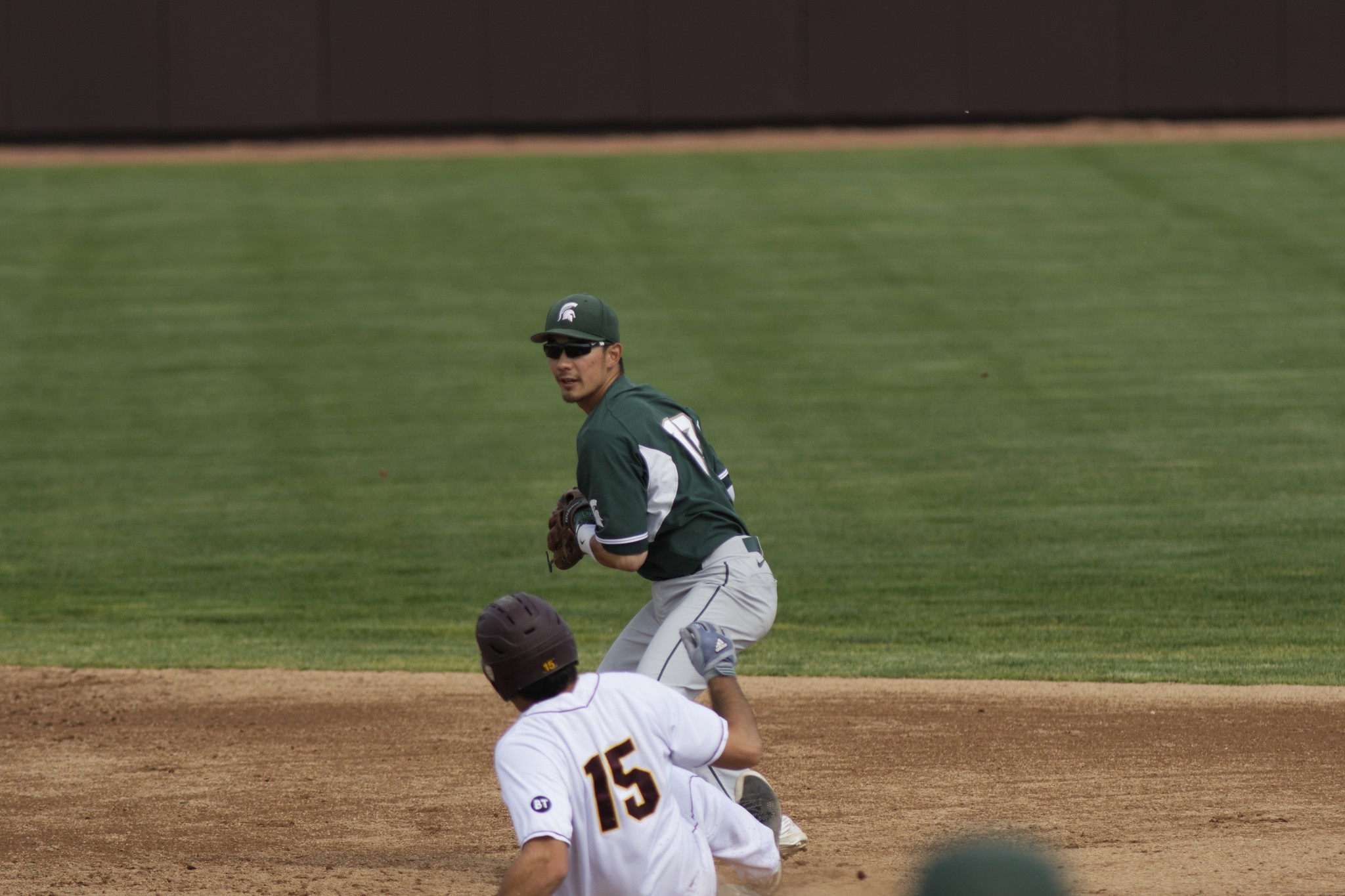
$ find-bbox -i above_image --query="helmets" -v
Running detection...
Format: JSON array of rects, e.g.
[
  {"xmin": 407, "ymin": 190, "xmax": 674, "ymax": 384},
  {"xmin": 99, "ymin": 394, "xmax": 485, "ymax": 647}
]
[{"xmin": 476, "ymin": 592, "xmax": 579, "ymax": 702}]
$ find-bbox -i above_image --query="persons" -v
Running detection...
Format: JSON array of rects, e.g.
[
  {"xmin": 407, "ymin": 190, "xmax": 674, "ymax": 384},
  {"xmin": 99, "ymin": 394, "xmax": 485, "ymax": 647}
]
[
  {"xmin": 475, "ymin": 593, "xmax": 783, "ymax": 896},
  {"xmin": 530, "ymin": 294, "xmax": 778, "ymax": 701},
  {"xmin": 919, "ymin": 838, "xmax": 1067, "ymax": 896}
]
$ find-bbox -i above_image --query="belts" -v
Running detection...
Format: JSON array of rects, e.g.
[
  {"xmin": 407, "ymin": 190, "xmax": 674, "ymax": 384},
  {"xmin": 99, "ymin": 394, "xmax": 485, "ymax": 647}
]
[{"xmin": 690, "ymin": 537, "xmax": 759, "ymax": 576}]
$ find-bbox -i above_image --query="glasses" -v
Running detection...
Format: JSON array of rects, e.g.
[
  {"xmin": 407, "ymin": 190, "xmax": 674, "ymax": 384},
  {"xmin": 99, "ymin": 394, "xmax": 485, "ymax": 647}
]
[{"xmin": 543, "ymin": 342, "xmax": 613, "ymax": 360}]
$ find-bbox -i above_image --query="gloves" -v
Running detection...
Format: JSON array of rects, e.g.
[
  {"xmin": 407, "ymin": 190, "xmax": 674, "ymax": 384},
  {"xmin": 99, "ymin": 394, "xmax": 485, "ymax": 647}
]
[{"xmin": 679, "ymin": 621, "xmax": 738, "ymax": 682}]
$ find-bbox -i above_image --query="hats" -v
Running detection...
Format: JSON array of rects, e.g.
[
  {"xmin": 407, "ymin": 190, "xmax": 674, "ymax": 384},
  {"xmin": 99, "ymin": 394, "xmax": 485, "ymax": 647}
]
[{"xmin": 530, "ymin": 293, "xmax": 620, "ymax": 344}]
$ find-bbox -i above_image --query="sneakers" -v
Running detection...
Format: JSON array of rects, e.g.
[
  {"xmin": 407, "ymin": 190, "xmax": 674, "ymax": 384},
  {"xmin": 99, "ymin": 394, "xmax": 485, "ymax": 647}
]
[
  {"xmin": 734, "ymin": 770, "xmax": 781, "ymax": 896},
  {"xmin": 779, "ymin": 814, "xmax": 808, "ymax": 861}
]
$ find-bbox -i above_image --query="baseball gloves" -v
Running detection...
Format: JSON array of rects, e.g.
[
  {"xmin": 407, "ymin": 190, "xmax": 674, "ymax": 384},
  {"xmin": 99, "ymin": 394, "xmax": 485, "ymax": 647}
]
[{"xmin": 543, "ymin": 487, "xmax": 588, "ymax": 576}]
